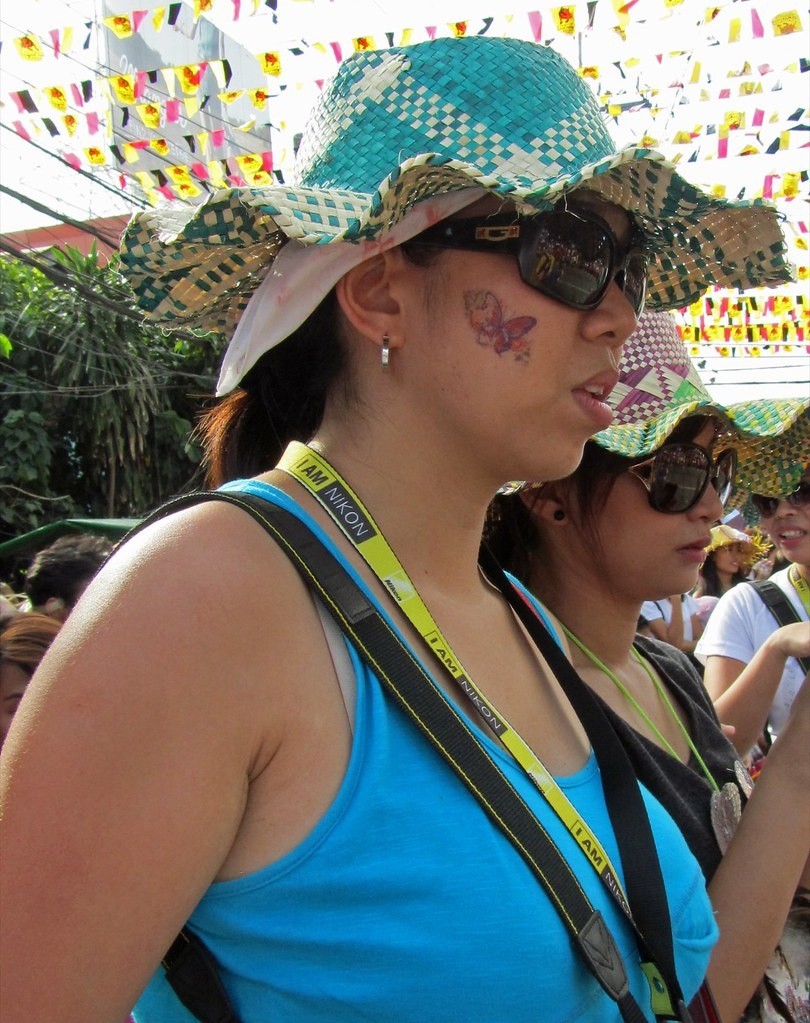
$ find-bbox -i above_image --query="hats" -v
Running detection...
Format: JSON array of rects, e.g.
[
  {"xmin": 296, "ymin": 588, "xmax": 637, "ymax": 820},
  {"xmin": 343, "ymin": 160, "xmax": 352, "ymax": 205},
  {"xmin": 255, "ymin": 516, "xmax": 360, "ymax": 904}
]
[
  {"xmin": 479, "ymin": 313, "xmax": 810, "ymax": 539},
  {"xmin": 121, "ymin": 37, "xmax": 794, "ymax": 339},
  {"xmin": 697, "ymin": 524, "xmax": 774, "ymax": 571}
]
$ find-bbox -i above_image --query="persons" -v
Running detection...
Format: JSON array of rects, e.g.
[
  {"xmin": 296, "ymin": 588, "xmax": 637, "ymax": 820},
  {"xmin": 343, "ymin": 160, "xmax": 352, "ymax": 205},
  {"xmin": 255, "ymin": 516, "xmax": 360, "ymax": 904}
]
[
  {"xmin": 0, "ymin": 35, "xmax": 801, "ymax": 1023},
  {"xmin": 26, "ymin": 533, "xmax": 115, "ymax": 624},
  {"xmin": 478, "ymin": 308, "xmax": 810, "ymax": 1023},
  {"xmin": 0, "ymin": 614, "xmax": 63, "ymax": 741}
]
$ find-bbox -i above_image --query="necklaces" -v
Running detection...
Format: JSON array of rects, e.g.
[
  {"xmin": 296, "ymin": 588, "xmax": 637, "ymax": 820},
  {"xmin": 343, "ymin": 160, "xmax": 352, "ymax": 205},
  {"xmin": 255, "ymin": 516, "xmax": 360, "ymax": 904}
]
[{"xmin": 555, "ymin": 617, "xmax": 720, "ymax": 796}]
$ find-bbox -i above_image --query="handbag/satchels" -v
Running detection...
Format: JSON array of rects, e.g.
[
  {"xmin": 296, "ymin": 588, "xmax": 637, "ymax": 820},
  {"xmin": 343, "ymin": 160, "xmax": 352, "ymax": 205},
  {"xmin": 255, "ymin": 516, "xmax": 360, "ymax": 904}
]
[{"xmin": 712, "ymin": 784, "xmax": 810, "ymax": 1023}]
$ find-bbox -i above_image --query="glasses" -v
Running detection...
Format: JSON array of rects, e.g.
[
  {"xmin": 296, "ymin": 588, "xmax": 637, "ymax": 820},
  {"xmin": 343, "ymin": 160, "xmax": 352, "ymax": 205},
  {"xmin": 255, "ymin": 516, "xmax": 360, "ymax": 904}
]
[
  {"xmin": 404, "ymin": 209, "xmax": 649, "ymax": 320},
  {"xmin": 751, "ymin": 482, "xmax": 810, "ymax": 517},
  {"xmin": 592, "ymin": 442, "xmax": 739, "ymax": 514}
]
